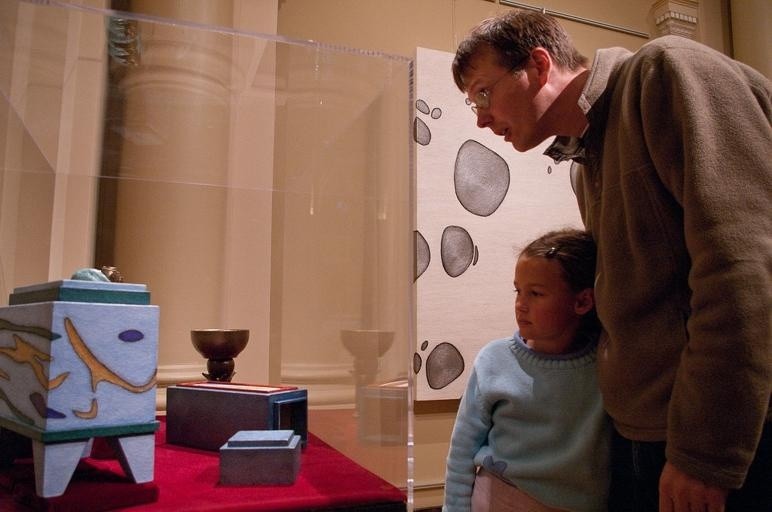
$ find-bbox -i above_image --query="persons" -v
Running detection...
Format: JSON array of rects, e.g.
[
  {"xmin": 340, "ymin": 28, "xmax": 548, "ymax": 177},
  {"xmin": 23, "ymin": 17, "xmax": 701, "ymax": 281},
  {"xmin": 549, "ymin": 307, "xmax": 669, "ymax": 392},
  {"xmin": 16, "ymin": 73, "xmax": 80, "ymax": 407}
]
[
  {"xmin": 452, "ymin": 11, "xmax": 772, "ymax": 512},
  {"xmin": 440, "ymin": 226, "xmax": 618, "ymax": 512}
]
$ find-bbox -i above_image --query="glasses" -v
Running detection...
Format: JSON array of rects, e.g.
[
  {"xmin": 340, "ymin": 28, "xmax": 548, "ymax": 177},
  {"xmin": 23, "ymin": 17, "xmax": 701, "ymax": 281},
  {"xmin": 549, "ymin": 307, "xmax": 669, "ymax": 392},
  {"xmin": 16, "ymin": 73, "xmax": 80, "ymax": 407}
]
[{"xmin": 465, "ymin": 69, "xmax": 512, "ymax": 114}]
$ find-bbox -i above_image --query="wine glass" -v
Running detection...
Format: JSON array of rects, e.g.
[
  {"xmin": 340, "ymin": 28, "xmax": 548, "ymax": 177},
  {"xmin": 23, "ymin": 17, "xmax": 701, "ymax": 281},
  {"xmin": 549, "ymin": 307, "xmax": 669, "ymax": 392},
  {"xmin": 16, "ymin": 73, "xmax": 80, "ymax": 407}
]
[{"xmin": 188, "ymin": 328, "xmax": 252, "ymax": 382}]
